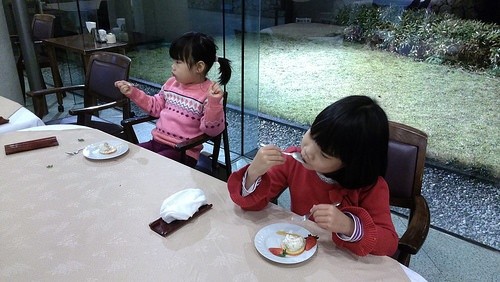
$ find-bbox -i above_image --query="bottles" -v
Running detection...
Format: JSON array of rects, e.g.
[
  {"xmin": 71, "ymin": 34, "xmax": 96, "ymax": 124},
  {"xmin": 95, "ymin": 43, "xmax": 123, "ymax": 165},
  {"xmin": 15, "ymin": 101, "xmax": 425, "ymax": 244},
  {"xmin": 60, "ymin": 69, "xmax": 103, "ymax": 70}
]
[{"xmin": 91, "ymin": 28, "xmax": 106, "ymax": 43}]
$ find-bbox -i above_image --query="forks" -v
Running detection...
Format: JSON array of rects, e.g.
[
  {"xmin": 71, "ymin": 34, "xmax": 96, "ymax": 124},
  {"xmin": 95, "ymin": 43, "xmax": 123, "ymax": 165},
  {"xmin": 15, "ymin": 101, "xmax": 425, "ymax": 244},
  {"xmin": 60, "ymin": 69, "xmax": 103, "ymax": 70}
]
[{"xmin": 291, "ymin": 202, "xmax": 340, "ymax": 222}]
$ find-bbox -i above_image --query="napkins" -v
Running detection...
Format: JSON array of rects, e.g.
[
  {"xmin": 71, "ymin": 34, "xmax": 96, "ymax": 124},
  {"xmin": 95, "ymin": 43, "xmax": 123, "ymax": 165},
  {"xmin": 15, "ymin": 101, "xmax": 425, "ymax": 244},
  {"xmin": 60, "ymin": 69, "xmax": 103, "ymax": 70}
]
[{"xmin": 159, "ymin": 187, "xmax": 206, "ymax": 223}]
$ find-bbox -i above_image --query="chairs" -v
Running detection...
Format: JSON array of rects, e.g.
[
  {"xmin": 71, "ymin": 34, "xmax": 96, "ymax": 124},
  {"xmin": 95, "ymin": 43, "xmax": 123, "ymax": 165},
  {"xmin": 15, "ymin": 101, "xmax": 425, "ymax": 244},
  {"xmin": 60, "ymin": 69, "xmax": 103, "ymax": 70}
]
[
  {"xmin": 26, "ymin": 52, "xmax": 133, "ymax": 139},
  {"xmin": 121, "ymin": 90, "xmax": 233, "ymax": 180},
  {"xmin": 276, "ymin": 120, "xmax": 431, "ymax": 269},
  {"xmin": 8, "ymin": 13, "xmax": 68, "ymax": 98}
]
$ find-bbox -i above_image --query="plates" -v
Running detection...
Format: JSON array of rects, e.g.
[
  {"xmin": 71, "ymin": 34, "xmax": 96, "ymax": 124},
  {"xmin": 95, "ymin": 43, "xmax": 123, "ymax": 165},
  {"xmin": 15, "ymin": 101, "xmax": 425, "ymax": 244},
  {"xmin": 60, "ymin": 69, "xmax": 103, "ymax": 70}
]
[
  {"xmin": 82, "ymin": 142, "xmax": 129, "ymax": 158},
  {"xmin": 254, "ymin": 223, "xmax": 317, "ymax": 264}
]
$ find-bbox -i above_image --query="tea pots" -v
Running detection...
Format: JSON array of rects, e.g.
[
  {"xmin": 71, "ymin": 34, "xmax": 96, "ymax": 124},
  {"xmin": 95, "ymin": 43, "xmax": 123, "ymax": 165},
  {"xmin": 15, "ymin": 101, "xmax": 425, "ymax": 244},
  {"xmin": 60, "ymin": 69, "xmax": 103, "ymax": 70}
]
[{"xmin": 103, "ymin": 33, "xmax": 117, "ymax": 44}]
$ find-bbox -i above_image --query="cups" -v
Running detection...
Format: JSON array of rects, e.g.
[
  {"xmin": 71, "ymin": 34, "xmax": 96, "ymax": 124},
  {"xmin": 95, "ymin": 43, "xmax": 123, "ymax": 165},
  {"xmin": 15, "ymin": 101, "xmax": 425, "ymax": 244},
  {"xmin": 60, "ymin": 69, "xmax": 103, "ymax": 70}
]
[{"xmin": 85, "ymin": 22, "xmax": 96, "ymax": 32}]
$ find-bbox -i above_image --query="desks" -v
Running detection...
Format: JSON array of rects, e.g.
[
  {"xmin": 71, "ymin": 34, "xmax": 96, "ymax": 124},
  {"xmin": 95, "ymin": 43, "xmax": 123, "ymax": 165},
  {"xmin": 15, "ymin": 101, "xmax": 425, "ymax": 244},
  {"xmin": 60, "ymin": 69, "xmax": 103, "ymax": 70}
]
[
  {"xmin": 0, "ymin": 120, "xmax": 428, "ymax": 282},
  {"xmin": 42, "ymin": 35, "xmax": 128, "ymax": 116},
  {"xmin": 0, "ymin": 95, "xmax": 46, "ymax": 132}
]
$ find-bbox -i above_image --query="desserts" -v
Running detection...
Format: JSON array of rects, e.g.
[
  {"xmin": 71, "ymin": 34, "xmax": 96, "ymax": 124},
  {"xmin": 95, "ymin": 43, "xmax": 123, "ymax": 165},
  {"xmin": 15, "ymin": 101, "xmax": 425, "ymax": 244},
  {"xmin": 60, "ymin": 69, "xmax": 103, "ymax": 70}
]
[
  {"xmin": 281, "ymin": 234, "xmax": 306, "ymax": 254},
  {"xmin": 100, "ymin": 142, "xmax": 117, "ymax": 154}
]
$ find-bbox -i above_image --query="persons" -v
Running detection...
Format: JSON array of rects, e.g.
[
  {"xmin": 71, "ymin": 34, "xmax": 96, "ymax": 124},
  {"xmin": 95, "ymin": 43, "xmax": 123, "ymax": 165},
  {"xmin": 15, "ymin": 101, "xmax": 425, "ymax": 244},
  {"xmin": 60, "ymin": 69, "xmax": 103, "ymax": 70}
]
[
  {"xmin": 112, "ymin": 31, "xmax": 232, "ymax": 169},
  {"xmin": 227, "ymin": 95, "xmax": 399, "ymax": 256}
]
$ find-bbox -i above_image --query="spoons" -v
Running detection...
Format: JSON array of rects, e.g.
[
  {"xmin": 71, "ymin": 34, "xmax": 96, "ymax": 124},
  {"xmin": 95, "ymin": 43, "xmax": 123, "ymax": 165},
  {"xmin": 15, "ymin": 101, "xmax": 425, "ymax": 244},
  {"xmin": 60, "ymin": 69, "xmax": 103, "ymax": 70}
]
[{"xmin": 260, "ymin": 142, "xmax": 305, "ymax": 165}]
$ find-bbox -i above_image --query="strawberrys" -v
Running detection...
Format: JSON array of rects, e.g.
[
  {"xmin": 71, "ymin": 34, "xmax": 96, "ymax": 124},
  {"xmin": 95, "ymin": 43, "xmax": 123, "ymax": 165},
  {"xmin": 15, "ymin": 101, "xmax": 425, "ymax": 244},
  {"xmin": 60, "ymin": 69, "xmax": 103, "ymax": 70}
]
[
  {"xmin": 305, "ymin": 236, "xmax": 317, "ymax": 251},
  {"xmin": 269, "ymin": 248, "xmax": 284, "ymax": 255}
]
